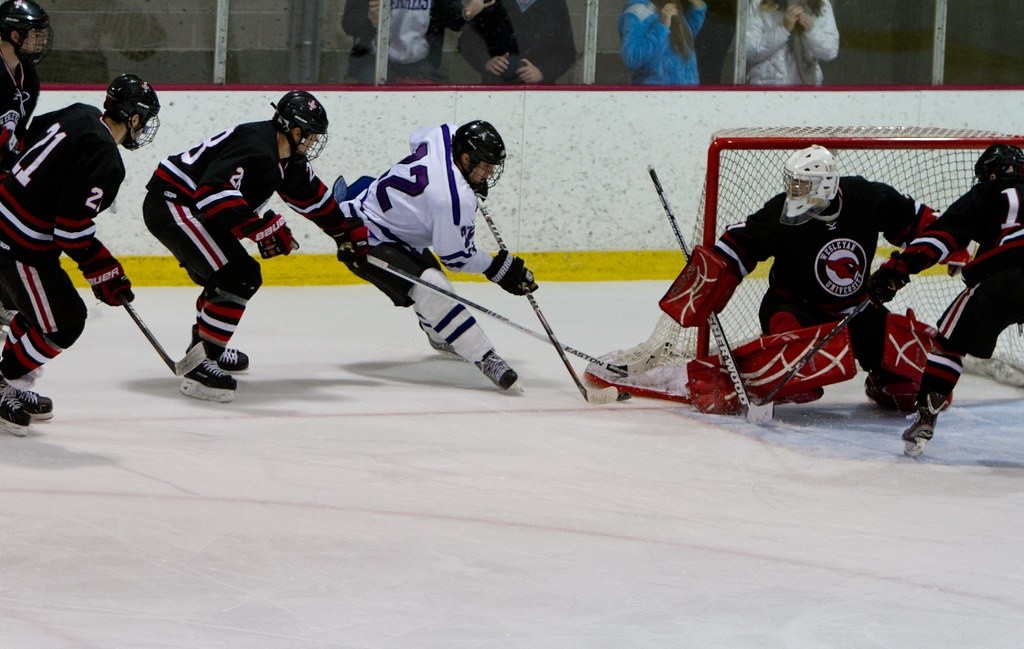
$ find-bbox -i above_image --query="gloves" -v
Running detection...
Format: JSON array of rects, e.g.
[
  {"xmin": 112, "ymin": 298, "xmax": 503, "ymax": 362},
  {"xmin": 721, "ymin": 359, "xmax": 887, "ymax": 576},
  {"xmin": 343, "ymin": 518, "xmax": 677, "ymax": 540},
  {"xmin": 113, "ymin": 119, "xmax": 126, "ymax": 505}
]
[
  {"xmin": 332, "ymin": 214, "xmax": 372, "ymax": 270},
  {"xmin": 241, "ymin": 209, "xmax": 299, "ymax": 260},
  {"xmin": 865, "ymin": 251, "xmax": 910, "ymax": 305},
  {"xmin": 484, "ymin": 250, "xmax": 539, "ymax": 297},
  {"xmin": 79, "ymin": 259, "xmax": 134, "ymax": 307}
]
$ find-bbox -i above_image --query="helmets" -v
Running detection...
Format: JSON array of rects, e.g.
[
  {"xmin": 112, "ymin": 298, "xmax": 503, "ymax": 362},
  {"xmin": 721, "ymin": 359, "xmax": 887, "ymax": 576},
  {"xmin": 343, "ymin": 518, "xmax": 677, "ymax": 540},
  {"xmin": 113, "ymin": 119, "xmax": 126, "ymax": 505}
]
[
  {"xmin": 452, "ymin": 120, "xmax": 507, "ymax": 191},
  {"xmin": 271, "ymin": 90, "xmax": 329, "ymax": 163},
  {"xmin": 778, "ymin": 146, "xmax": 839, "ymax": 223},
  {"xmin": 104, "ymin": 74, "xmax": 161, "ymax": 152},
  {"xmin": 975, "ymin": 144, "xmax": 1024, "ymax": 182},
  {"xmin": 0, "ymin": 0, "xmax": 54, "ymax": 67}
]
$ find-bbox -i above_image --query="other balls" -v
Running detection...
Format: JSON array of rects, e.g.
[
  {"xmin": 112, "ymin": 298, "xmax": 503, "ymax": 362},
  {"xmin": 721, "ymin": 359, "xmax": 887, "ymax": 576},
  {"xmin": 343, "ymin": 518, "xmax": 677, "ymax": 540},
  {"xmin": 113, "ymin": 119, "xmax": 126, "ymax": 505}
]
[{"xmin": 617, "ymin": 392, "xmax": 632, "ymax": 402}]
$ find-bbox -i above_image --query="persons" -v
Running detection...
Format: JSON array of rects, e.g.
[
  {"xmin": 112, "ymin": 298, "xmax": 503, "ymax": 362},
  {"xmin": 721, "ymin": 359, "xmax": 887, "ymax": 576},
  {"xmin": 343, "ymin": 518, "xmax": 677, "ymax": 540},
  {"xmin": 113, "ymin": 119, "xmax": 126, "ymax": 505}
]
[
  {"xmin": 618, "ymin": 0, "xmax": 706, "ymax": 85},
  {"xmin": 334, "ymin": 121, "xmax": 535, "ymax": 394},
  {"xmin": 727, "ymin": 1, "xmax": 839, "ymax": 87},
  {"xmin": 659, "ymin": 144, "xmax": 970, "ymax": 414},
  {"xmin": 0, "ymin": 0, "xmax": 45, "ymax": 341},
  {"xmin": 458, "ymin": 0, "xmax": 576, "ymax": 86},
  {"xmin": 341, "ymin": 0, "xmax": 496, "ymax": 86},
  {"xmin": 34, "ymin": 2, "xmax": 166, "ymax": 86},
  {"xmin": 142, "ymin": 90, "xmax": 369, "ymax": 402},
  {"xmin": 865, "ymin": 144, "xmax": 1024, "ymax": 457},
  {"xmin": 0, "ymin": 73, "xmax": 161, "ymax": 437}
]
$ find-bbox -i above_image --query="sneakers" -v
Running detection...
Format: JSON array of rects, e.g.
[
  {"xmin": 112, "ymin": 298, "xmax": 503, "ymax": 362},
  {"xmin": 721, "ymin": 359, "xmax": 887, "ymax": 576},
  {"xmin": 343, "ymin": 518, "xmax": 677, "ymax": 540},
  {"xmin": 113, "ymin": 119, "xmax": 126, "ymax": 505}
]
[
  {"xmin": 186, "ymin": 324, "xmax": 251, "ymax": 376},
  {"xmin": 901, "ymin": 395, "xmax": 947, "ymax": 458},
  {"xmin": 178, "ymin": 358, "xmax": 238, "ymax": 402},
  {"xmin": 0, "ymin": 381, "xmax": 31, "ymax": 436},
  {"xmin": 0, "ymin": 373, "xmax": 54, "ymax": 420},
  {"xmin": 423, "ymin": 333, "xmax": 466, "ymax": 361},
  {"xmin": 475, "ymin": 346, "xmax": 525, "ymax": 396}
]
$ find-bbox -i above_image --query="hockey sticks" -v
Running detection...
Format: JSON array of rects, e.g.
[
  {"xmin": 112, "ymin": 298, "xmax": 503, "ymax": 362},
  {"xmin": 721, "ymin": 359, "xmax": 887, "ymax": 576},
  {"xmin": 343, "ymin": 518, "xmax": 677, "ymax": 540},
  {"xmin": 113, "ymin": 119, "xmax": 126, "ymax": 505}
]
[
  {"xmin": 339, "ymin": 243, "xmax": 674, "ymax": 378},
  {"xmin": 478, "ymin": 197, "xmax": 620, "ymax": 404},
  {"xmin": 647, "ymin": 162, "xmax": 777, "ymax": 427},
  {"xmin": 117, "ymin": 292, "xmax": 210, "ymax": 379},
  {"xmin": 756, "ymin": 298, "xmax": 874, "ymax": 405}
]
